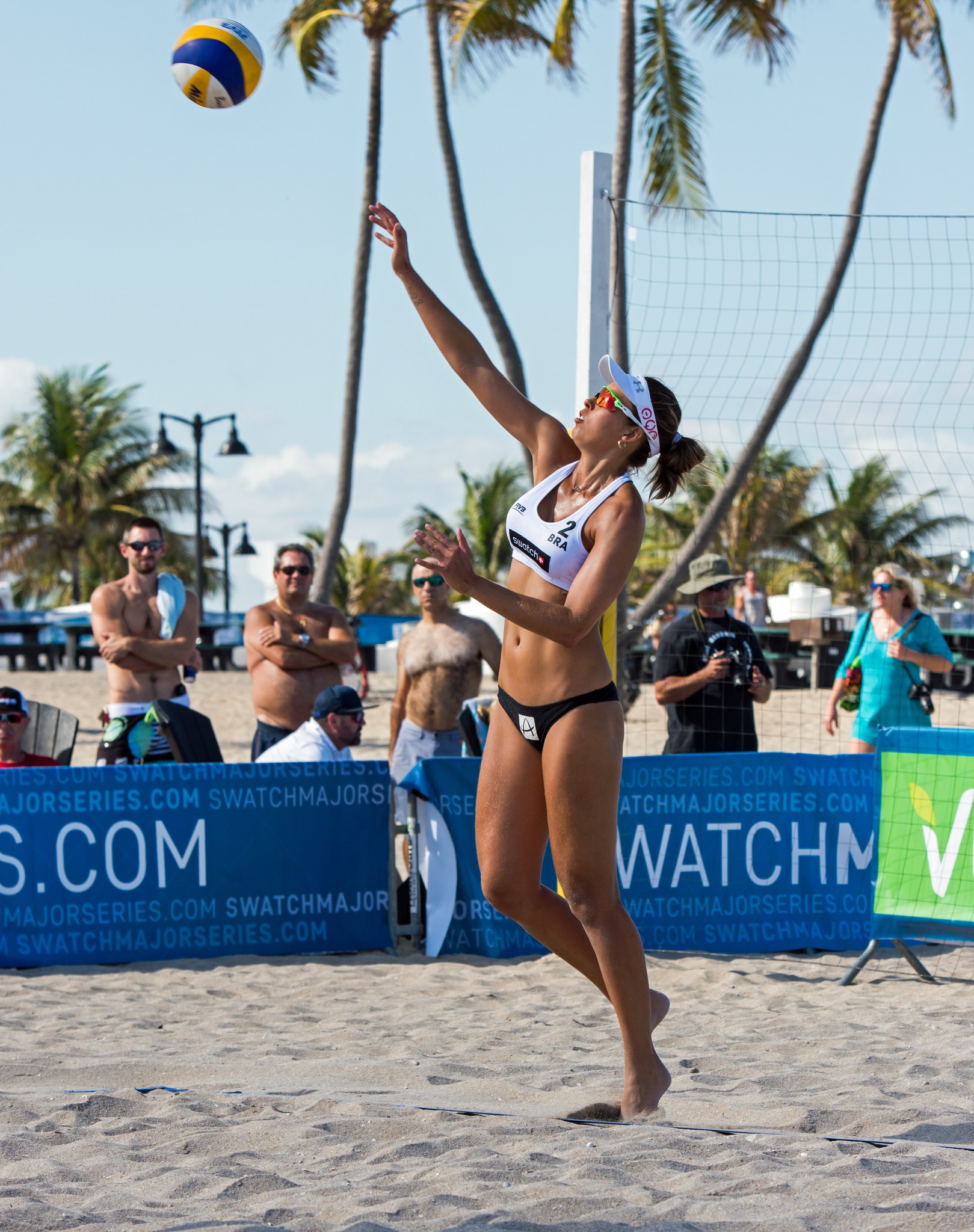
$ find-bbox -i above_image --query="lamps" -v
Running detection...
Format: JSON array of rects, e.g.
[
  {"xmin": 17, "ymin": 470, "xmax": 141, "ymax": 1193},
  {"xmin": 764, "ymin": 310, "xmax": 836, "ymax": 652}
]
[
  {"xmin": 234, "ymin": 522, "xmax": 257, "ymax": 555},
  {"xmin": 148, "ymin": 412, "xmax": 179, "ymax": 458},
  {"xmin": 202, "ymin": 524, "xmax": 218, "ymax": 560},
  {"xmin": 219, "ymin": 414, "xmax": 247, "ymax": 456}
]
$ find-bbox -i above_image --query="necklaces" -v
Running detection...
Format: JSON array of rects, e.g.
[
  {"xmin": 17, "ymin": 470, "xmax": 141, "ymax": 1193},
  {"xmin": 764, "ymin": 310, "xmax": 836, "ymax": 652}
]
[
  {"xmin": 573, "ymin": 469, "xmax": 626, "ymax": 502},
  {"xmin": 275, "ymin": 596, "xmax": 310, "ymax": 628},
  {"xmin": 883, "ymin": 627, "xmax": 889, "ymax": 632}
]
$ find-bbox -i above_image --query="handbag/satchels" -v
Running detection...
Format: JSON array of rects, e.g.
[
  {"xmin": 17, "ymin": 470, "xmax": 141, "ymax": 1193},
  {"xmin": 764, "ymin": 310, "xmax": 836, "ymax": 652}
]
[{"xmin": 840, "ymin": 609, "xmax": 874, "ymax": 712}]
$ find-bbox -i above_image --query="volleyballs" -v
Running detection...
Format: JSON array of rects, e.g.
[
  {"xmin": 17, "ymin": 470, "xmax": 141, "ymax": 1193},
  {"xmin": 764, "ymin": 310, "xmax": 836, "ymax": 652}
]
[{"xmin": 170, "ymin": 16, "xmax": 262, "ymax": 110}]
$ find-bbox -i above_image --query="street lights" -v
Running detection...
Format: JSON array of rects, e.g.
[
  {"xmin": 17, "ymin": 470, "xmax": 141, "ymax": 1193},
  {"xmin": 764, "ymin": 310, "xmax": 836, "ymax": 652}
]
[{"xmin": 152, "ymin": 411, "xmax": 258, "ymax": 624}]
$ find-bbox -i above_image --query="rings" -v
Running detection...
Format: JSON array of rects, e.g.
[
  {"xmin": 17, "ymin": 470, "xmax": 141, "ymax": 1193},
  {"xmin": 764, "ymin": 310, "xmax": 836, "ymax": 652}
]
[
  {"xmin": 712, "ymin": 653, "xmax": 718, "ymax": 659},
  {"xmin": 268, "ymin": 633, "xmax": 272, "ymax": 638}
]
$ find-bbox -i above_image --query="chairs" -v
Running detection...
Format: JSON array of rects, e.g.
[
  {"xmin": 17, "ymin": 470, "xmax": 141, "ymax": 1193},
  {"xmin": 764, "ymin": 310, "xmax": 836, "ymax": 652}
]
[
  {"xmin": 23, "ymin": 698, "xmax": 81, "ymax": 767},
  {"xmin": 150, "ymin": 696, "xmax": 225, "ymax": 762},
  {"xmin": 454, "ymin": 695, "xmax": 497, "ymax": 757}
]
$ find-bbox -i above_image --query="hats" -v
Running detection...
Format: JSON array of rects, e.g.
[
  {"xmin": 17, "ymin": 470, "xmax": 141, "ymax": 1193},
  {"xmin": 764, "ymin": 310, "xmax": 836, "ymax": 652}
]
[
  {"xmin": 0, "ymin": 686, "xmax": 29, "ymax": 717},
  {"xmin": 677, "ymin": 553, "xmax": 748, "ymax": 595},
  {"xmin": 313, "ymin": 685, "xmax": 379, "ymax": 720},
  {"xmin": 598, "ymin": 353, "xmax": 660, "ymax": 458}
]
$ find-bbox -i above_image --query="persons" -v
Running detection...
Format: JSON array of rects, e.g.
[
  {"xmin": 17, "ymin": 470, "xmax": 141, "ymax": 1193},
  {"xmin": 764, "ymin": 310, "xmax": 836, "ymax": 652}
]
[
  {"xmin": 823, "ymin": 562, "xmax": 955, "ymax": 754},
  {"xmin": 0, "ymin": 687, "xmax": 62, "ymax": 768},
  {"xmin": 653, "ymin": 553, "xmax": 775, "ymax": 755},
  {"xmin": 388, "ymin": 557, "xmax": 502, "ymax": 941},
  {"xmin": 733, "ymin": 570, "xmax": 774, "ymax": 627},
  {"xmin": 90, "ymin": 516, "xmax": 203, "ymax": 766},
  {"xmin": 642, "ymin": 603, "xmax": 677, "ymax": 651},
  {"xmin": 254, "ymin": 686, "xmax": 379, "ymax": 763},
  {"xmin": 243, "ymin": 543, "xmax": 357, "ymax": 763},
  {"xmin": 366, "ymin": 201, "xmax": 706, "ymax": 1125}
]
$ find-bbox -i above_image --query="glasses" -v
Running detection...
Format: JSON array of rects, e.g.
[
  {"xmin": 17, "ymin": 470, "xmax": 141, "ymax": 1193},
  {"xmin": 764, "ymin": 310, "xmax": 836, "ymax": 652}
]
[
  {"xmin": 870, "ymin": 582, "xmax": 905, "ymax": 592},
  {"xmin": 339, "ymin": 712, "xmax": 364, "ymax": 723},
  {"xmin": 594, "ymin": 386, "xmax": 655, "ymax": 443},
  {"xmin": 0, "ymin": 715, "xmax": 20, "ymax": 723},
  {"xmin": 707, "ymin": 582, "xmax": 730, "ymax": 592},
  {"xmin": 124, "ymin": 539, "xmax": 162, "ymax": 551},
  {"xmin": 279, "ymin": 565, "xmax": 310, "ymax": 575},
  {"xmin": 411, "ymin": 575, "xmax": 443, "ymax": 587}
]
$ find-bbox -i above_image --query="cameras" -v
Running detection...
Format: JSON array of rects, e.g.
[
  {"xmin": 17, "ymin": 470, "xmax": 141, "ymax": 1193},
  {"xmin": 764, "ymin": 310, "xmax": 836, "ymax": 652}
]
[
  {"xmin": 724, "ymin": 648, "xmax": 753, "ymax": 688},
  {"xmin": 907, "ymin": 683, "xmax": 935, "ymax": 715}
]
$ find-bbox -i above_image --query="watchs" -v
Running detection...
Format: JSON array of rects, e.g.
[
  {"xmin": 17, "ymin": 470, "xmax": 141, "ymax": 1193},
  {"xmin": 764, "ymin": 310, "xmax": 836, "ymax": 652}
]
[{"xmin": 298, "ymin": 633, "xmax": 311, "ymax": 648}]
